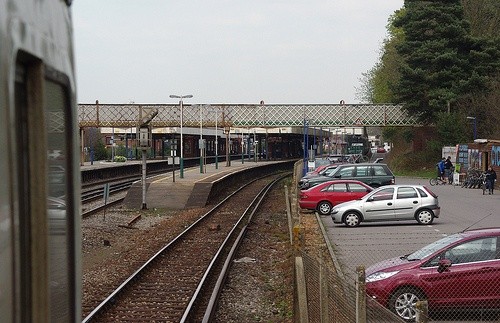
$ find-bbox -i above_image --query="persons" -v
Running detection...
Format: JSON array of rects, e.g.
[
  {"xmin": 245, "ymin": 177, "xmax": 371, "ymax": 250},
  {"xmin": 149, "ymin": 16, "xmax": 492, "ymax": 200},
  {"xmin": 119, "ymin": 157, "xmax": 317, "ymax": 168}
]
[
  {"xmin": 484, "ymin": 166, "xmax": 497, "ymax": 195},
  {"xmin": 438, "ymin": 156, "xmax": 454, "ymax": 184},
  {"xmin": 460, "ymin": 164, "xmax": 468, "ymax": 179}
]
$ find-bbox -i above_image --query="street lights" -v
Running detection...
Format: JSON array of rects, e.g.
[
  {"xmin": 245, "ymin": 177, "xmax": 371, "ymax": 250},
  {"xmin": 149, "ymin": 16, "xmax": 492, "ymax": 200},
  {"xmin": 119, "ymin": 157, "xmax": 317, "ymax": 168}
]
[
  {"xmin": 191, "ymin": 103, "xmax": 211, "ymax": 173},
  {"xmin": 207, "ymin": 110, "xmax": 225, "ymax": 169},
  {"xmin": 169, "ymin": 95, "xmax": 193, "ymax": 178},
  {"xmin": 238, "ymin": 126, "xmax": 256, "ymax": 164}
]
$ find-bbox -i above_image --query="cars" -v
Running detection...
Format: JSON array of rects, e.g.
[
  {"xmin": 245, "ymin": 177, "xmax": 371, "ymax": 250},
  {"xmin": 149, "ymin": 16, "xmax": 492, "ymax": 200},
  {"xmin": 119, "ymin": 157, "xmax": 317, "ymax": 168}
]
[
  {"xmin": 354, "ymin": 227, "xmax": 500, "ymax": 323},
  {"xmin": 298, "ymin": 180, "xmax": 401, "ymax": 215},
  {"xmin": 331, "ymin": 185, "xmax": 440, "ymax": 228}
]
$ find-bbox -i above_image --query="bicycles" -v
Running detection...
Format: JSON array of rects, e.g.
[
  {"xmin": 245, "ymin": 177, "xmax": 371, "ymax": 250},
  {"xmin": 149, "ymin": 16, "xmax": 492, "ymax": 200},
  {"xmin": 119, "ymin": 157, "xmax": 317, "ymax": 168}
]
[{"xmin": 429, "ymin": 167, "xmax": 489, "ymax": 195}]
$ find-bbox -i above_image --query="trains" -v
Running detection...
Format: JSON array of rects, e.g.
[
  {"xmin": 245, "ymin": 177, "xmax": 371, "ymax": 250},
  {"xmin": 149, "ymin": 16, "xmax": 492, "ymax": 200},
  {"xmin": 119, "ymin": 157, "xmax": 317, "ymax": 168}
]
[{"xmin": 0, "ymin": 0, "xmax": 82, "ymax": 323}]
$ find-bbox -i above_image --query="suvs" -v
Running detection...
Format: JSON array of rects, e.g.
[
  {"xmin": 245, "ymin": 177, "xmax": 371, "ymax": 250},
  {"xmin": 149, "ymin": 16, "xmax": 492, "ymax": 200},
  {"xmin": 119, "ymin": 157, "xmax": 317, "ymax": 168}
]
[{"xmin": 298, "ymin": 158, "xmax": 395, "ymax": 189}]
